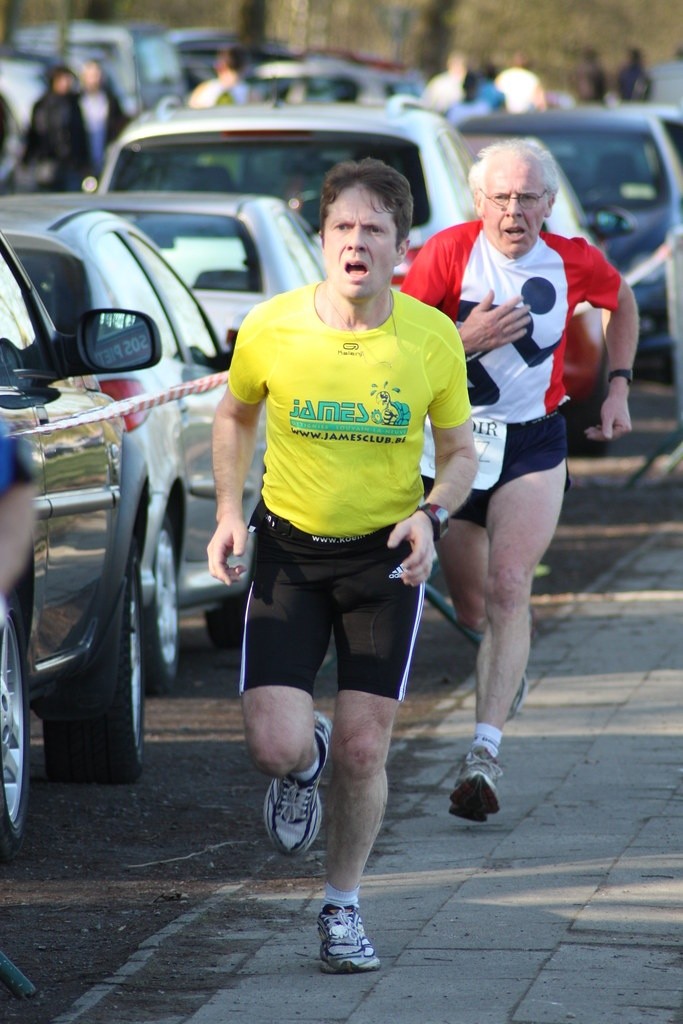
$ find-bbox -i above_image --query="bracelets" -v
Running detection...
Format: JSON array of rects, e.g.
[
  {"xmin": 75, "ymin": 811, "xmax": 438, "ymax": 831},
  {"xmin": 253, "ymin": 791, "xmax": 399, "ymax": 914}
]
[{"xmin": 608, "ymin": 369, "xmax": 633, "ymax": 383}]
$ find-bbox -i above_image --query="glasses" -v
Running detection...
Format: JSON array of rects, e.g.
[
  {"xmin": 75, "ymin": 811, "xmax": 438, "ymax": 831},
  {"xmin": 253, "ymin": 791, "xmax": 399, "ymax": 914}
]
[{"xmin": 479, "ymin": 190, "xmax": 547, "ymax": 209}]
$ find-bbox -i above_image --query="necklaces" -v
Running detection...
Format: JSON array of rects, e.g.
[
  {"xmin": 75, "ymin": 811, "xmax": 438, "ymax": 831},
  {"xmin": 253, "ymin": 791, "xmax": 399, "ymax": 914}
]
[{"xmin": 324, "ymin": 292, "xmax": 397, "ymax": 341}]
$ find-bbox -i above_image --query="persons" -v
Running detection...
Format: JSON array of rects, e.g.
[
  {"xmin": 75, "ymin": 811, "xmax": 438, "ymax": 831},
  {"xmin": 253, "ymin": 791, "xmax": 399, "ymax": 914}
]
[
  {"xmin": 420, "ymin": 49, "xmax": 547, "ymax": 127},
  {"xmin": 207, "ymin": 158, "xmax": 479, "ymax": 971},
  {"xmin": 0, "ymin": 421, "xmax": 41, "ymax": 604},
  {"xmin": 617, "ymin": 49, "xmax": 651, "ymax": 102},
  {"xmin": 400, "ymin": 137, "xmax": 640, "ymax": 821},
  {"xmin": 74, "ymin": 62, "xmax": 124, "ymax": 172},
  {"xmin": 574, "ymin": 48, "xmax": 605, "ymax": 105},
  {"xmin": 190, "ymin": 44, "xmax": 261, "ymax": 107},
  {"xmin": 21, "ymin": 66, "xmax": 96, "ymax": 193}
]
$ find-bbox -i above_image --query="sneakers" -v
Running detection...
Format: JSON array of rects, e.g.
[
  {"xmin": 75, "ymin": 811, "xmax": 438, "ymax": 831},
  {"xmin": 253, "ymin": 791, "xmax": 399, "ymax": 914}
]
[
  {"xmin": 505, "ymin": 672, "xmax": 528, "ymax": 721},
  {"xmin": 448, "ymin": 748, "xmax": 503, "ymax": 822},
  {"xmin": 263, "ymin": 712, "xmax": 333, "ymax": 857},
  {"xmin": 317, "ymin": 903, "xmax": 381, "ymax": 974}
]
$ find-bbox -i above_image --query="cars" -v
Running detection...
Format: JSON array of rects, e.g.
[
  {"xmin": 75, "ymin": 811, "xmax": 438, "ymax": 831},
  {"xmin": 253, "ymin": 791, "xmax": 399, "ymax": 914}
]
[
  {"xmin": 0, "ymin": 199, "xmax": 271, "ymax": 700},
  {"xmin": 0, "ymin": 228, "xmax": 164, "ymax": 859},
  {"xmin": 0, "ymin": 18, "xmax": 683, "ymax": 393}
]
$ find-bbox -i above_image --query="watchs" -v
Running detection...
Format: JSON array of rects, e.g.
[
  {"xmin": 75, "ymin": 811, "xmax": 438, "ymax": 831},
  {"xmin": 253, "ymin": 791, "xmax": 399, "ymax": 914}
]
[{"xmin": 415, "ymin": 503, "xmax": 449, "ymax": 541}]
